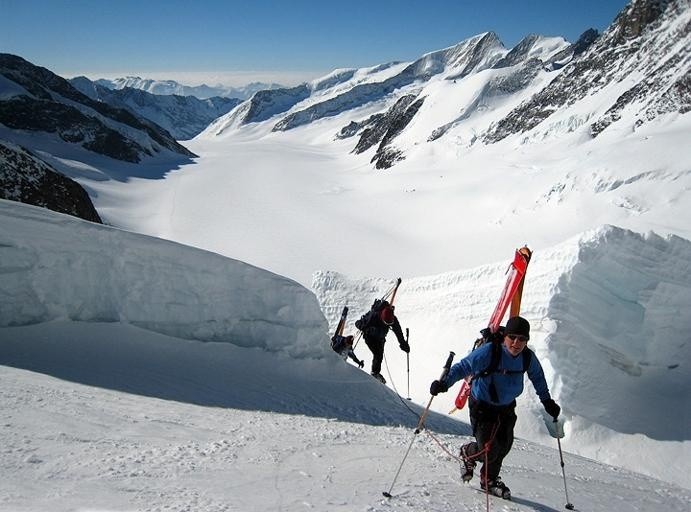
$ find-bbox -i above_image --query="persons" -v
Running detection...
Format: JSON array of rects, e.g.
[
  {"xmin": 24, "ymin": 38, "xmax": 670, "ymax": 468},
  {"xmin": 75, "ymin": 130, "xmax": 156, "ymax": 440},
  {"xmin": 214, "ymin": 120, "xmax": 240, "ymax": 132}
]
[
  {"xmin": 430, "ymin": 316, "xmax": 561, "ymax": 499},
  {"xmin": 332, "ymin": 334, "xmax": 365, "ymax": 368},
  {"xmin": 356, "ymin": 306, "xmax": 411, "ymax": 383}
]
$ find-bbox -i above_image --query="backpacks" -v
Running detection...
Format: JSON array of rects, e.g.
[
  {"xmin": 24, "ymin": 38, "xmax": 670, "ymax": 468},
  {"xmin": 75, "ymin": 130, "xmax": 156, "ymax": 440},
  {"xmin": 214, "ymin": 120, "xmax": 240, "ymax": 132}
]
[{"xmin": 472, "ymin": 326, "xmax": 505, "ymax": 377}]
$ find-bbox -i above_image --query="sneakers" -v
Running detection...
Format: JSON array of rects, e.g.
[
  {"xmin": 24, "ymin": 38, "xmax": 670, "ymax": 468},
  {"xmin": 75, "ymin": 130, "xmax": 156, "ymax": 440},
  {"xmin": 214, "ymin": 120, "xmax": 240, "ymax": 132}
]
[
  {"xmin": 371, "ymin": 371, "xmax": 386, "ymax": 384},
  {"xmin": 481, "ymin": 477, "xmax": 510, "ymax": 499},
  {"xmin": 460, "ymin": 445, "xmax": 474, "ymax": 480}
]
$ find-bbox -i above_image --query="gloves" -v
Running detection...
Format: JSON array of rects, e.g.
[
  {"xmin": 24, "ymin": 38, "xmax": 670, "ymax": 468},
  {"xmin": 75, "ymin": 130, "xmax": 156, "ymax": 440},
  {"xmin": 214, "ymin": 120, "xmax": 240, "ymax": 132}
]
[
  {"xmin": 357, "ymin": 361, "xmax": 364, "ymax": 367},
  {"xmin": 400, "ymin": 341, "xmax": 410, "ymax": 352},
  {"xmin": 541, "ymin": 398, "xmax": 560, "ymax": 416},
  {"xmin": 430, "ymin": 380, "xmax": 448, "ymax": 395}
]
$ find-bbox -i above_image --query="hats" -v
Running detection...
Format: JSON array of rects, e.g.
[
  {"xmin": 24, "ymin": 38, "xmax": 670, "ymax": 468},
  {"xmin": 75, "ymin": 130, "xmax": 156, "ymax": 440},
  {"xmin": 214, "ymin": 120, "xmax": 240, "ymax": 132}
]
[
  {"xmin": 382, "ymin": 308, "xmax": 393, "ymax": 323},
  {"xmin": 346, "ymin": 336, "xmax": 354, "ymax": 345},
  {"xmin": 505, "ymin": 317, "xmax": 529, "ymax": 338}
]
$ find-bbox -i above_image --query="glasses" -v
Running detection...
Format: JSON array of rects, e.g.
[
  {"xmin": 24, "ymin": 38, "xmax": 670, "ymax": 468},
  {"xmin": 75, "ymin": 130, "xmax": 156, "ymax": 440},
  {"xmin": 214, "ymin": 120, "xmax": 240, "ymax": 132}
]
[{"xmin": 508, "ymin": 335, "xmax": 528, "ymax": 341}]
